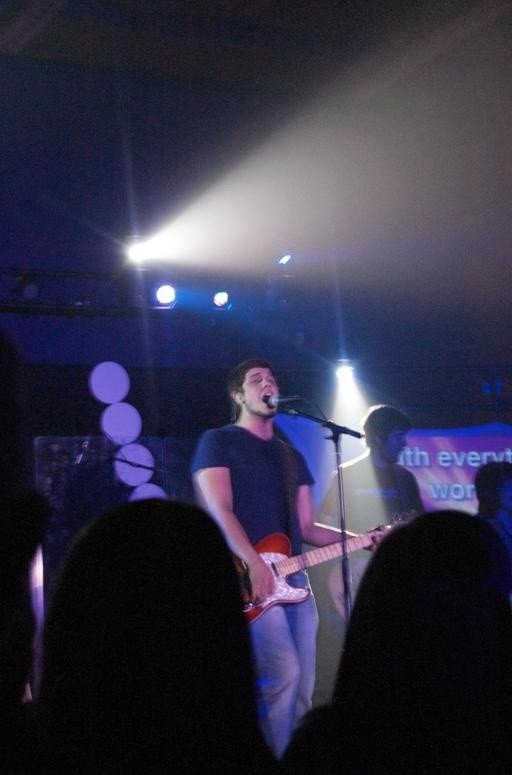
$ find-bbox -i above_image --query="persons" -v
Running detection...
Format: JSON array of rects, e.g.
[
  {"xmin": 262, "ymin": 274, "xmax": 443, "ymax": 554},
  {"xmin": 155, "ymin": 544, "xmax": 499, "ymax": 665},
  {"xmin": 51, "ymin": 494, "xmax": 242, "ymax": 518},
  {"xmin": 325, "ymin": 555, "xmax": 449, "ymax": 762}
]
[
  {"xmin": 474, "ymin": 460, "xmax": 512, "ymax": 550},
  {"xmin": 278, "ymin": 509, "xmax": 512, "ymax": 775},
  {"xmin": 322, "ymin": 404, "xmax": 427, "ymax": 535},
  {"xmin": 0, "ymin": 499, "xmax": 280, "ymax": 775},
  {"xmin": 191, "ymin": 356, "xmax": 314, "ymax": 763}
]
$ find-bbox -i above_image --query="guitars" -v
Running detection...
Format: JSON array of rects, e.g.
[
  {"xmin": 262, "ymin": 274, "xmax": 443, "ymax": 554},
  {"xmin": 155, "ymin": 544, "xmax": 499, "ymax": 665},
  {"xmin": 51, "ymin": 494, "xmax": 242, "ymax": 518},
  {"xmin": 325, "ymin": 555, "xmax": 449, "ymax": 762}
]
[{"xmin": 232, "ymin": 508, "xmax": 423, "ymax": 624}]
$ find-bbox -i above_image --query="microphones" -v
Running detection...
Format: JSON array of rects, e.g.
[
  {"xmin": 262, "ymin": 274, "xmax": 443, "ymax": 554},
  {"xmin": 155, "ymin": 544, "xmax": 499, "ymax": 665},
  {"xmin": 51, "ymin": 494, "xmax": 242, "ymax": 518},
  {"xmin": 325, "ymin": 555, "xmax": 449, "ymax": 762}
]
[
  {"xmin": 74, "ymin": 439, "xmax": 91, "ymax": 466},
  {"xmin": 265, "ymin": 394, "xmax": 305, "ymax": 408}
]
